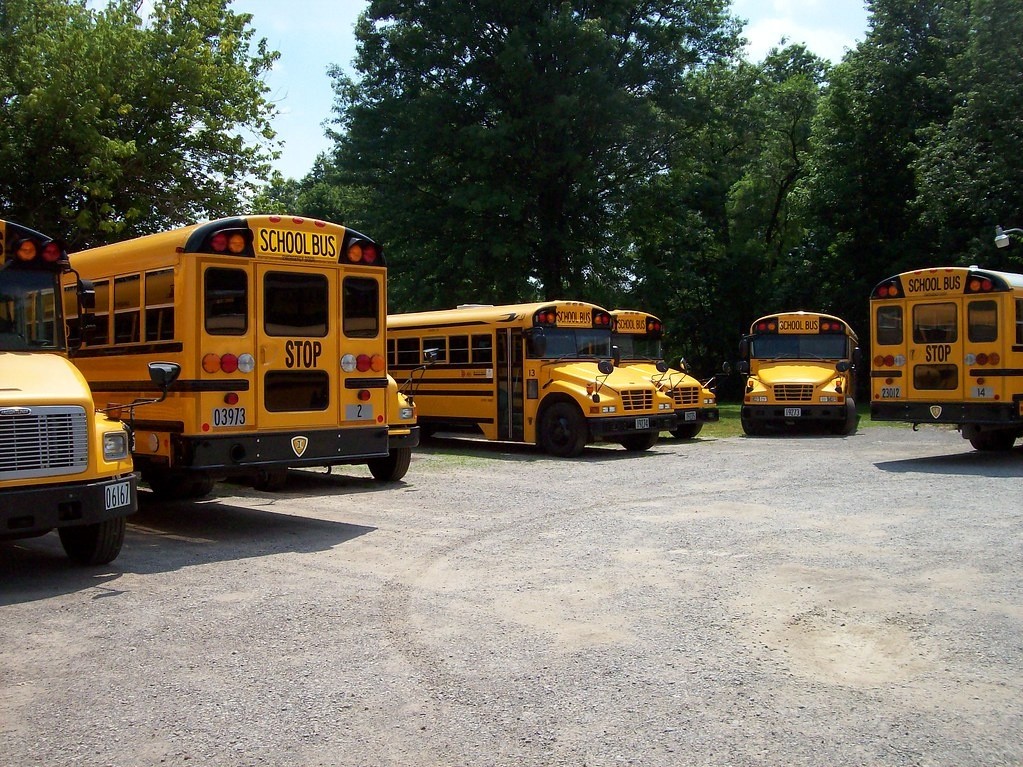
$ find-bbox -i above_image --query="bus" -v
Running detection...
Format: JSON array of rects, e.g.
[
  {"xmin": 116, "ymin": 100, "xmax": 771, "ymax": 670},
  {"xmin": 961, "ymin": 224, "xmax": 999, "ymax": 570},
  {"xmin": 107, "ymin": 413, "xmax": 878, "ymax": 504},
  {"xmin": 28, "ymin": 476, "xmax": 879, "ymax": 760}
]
[
  {"xmin": 1, "ymin": 214, "xmax": 442, "ymax": 502},
  {"xmin": 499, "ymin": 329, "xmax": 525, "ymax": 411},
  {"xmin": 388, "ymin": 300, "xmax": 691, "ymax": 457},
  {"xmin": 1, "ymin": 221, "xmax": 181, "ymax": 566},
  {"xmin": 736, "ymin": 311, "xmax": 860, "ymax": 437},
  {"xmin": 608, "ymin": 309, "xmax": 733, "ymax": 442},
  {"xmin": 869, "ymin": 265, "xmax": 1022, "ymax": 456}
]
